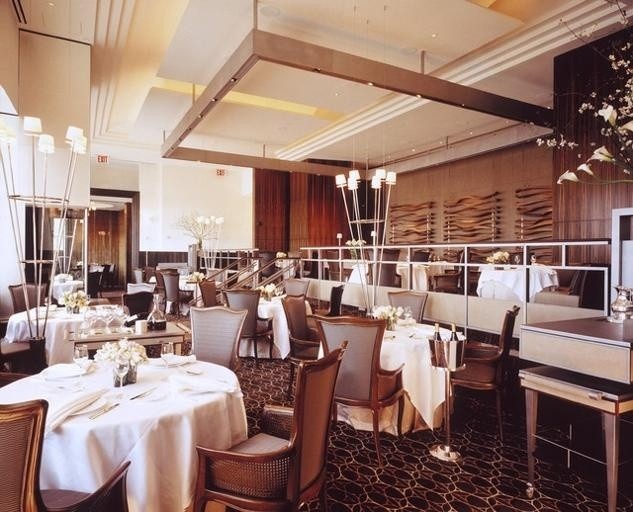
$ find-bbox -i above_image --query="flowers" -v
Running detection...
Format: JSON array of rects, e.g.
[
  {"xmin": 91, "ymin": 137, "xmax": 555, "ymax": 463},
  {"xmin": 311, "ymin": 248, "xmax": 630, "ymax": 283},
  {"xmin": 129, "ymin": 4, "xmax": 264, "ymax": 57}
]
[
  {"xmin": 374, "ymin": 304, "xmax": 404, "ymax": 322},
  {"xmin": 487, "ymin": 251, "xmax": 510, "ymax": 262},
  {"xmin": 96, "ymin": 336, "xmax": 149, "ymax": 368},
  {"xmin": 63, "ymin": 290, "xmax": 90, "ymax": 307}
]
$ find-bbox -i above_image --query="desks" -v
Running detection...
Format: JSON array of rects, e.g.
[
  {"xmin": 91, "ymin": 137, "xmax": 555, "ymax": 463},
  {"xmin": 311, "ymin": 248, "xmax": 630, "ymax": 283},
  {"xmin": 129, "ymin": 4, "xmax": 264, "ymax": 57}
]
[{"xmin": 518, "ymin": 366, "xmax": 633, "ymax": 512}]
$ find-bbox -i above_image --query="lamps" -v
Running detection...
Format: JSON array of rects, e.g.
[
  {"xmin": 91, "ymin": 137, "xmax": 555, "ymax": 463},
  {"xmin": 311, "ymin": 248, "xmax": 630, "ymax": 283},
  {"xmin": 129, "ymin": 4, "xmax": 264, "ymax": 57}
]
[
  {"xmin": 334, "ymin": 167, "xmax": 397, "ymax": 313},
  {"xmin": 198, "ymin": 215, "xmax": 226, "ymax": 276},
  {"xmin": 0, "ymin": 116, "xmax": 86, "ymax": 369}
]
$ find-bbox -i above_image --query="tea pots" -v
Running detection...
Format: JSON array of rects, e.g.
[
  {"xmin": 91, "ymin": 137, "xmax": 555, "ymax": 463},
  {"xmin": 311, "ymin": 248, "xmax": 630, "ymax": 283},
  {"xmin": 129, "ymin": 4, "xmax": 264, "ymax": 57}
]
[
  {"xmin": 147, "ymin": 294, "xmax": 167, "ymax": 330},
  {"xmin": 610, "ymin": 285, "xmax": 633, "ymax": 320}
]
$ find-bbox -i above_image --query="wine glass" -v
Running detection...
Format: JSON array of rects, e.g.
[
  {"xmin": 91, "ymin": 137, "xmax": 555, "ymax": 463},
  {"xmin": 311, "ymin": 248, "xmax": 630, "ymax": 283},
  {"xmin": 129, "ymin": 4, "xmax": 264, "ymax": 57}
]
[
  {"xmin": 113, "ymin": 305, "xmax": 130, "ymax": 334},
  {"xmin": 72, "ymin": 344, "xmax": 88, "ymax": 387},
  {"xmin": 84, "ymin": 307, "xmax": 97, "ymax": 337},
  {"xmin": 99, "ymin": 306, "xmax": 113, "ymax": 335},
  {"xmin": 161, "ymin": 343, "xmax": 174, "ymax": 378}
]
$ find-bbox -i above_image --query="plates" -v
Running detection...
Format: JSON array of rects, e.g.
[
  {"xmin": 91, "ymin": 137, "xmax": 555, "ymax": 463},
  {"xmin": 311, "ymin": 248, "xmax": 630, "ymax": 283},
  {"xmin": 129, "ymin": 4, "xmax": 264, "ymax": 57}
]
[
  {"xmin": 42, "ymin": 363, "xmax": 93, "ymax": 379},
  {"xmin": 70, "ymin": 395, "xmax": 108, "ymax": 418}
]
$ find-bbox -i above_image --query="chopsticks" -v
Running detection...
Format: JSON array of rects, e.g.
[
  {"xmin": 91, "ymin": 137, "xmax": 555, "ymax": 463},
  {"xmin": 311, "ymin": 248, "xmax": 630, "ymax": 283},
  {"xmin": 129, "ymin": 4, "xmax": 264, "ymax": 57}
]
[{"xmin": 88, "ymin": 401, "xmax": 118, "ymax": 421}]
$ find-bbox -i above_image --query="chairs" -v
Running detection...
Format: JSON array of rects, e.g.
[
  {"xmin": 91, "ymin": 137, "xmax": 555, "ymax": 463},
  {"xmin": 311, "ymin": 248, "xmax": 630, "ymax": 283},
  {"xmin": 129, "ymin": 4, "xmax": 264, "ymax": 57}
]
[
  {"xmin": 435, "ymin": 249, "xmax": 474, "ymax": 293},
  {"xmin": 327, "ymin": 250, "xmax": 345, "ymax": 282},
  {"xmin": 91, "ymin": 265, "xmax": 106, "ymax": 284},
  {"xmin": 220, "ymin": 289, "xmax": 274, "ymax": 368},
  {"xmin": 280, "ymin": 294, "xmax": 322, "ymax": 399},
  {"xmin": 388, "ymin": 290, "xmax": 429, "ymax": 323},
  {"xmin": 326, "ymin": 285, "xmax": 344, "ymax": 316},
  {"xmin": 312, "ymin": 313, "xmax": 407, "ymax": 469},
  {"xmin": 366, "ymin": 248, "xmax": 401, "ymax": 285},
  {"xmin": 412, "ymin": 250, "xmax": 431, "ymax": 262},
  {"xmin": 191, "ymin": 305, "xmax": 249, "ymax": 371},
  {"xmin": 123, "ymin": 291, "xmax": 153, "ymax": 317},
  {"xmin": 535, "ymin": 262, "xmax": 608, "ymax": 310},
  {"xmin": 192, "ymin": 340, "xmax": 349, "ymax": 511},
  {"xmin": 87, "ymin": 270, "xmax": 103, "ymax": 299},
  {"xmin": 0, "ymin": 399, "xmax": 132, "ymax": 512},
  {"xmin": 162, "ymin": 273, "xmax": 194, "ymax": 315},
  {"xmin": 449, "ymin": 304, "xmax": 520, "ymax": 441},
  {"xmin": 8, "ymin": 282, "xmax": 46, "ymax": 313},
  {"xmin": 126, "ymin": 283, "xmax": 156, "ymax": 295},
  {"xmin": 286, "ymin": 279, "xmax": 311, "ymax": 295},
  {"xmin": 199, "ymin": 278, "xmax": 216, "ymax": 307},
  {"xmin": 153, "ymin": 269, "xmax": 165, "ymax": 298},
  {"xmin": 100, "ymin": 263, "xmax": 111, "ymax": 280}
]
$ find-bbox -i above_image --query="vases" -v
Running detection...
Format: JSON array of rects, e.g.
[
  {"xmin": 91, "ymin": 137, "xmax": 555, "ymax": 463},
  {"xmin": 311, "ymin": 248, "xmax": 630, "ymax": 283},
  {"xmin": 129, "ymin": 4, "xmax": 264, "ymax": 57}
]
[
  {"xmin": 73, "ymin": 305, "xmax": 79, "ymax": 315},
  {"xmin": 386, "ymin": 319, "xmax": 395, "ymax": 331},
  {"xmin": 114, "ymin": 366, "xmax": 138, "ymax": 388},
  {"xmin": 494, "ymin": 262, "xmax": 504, "ymax": 271}
]
